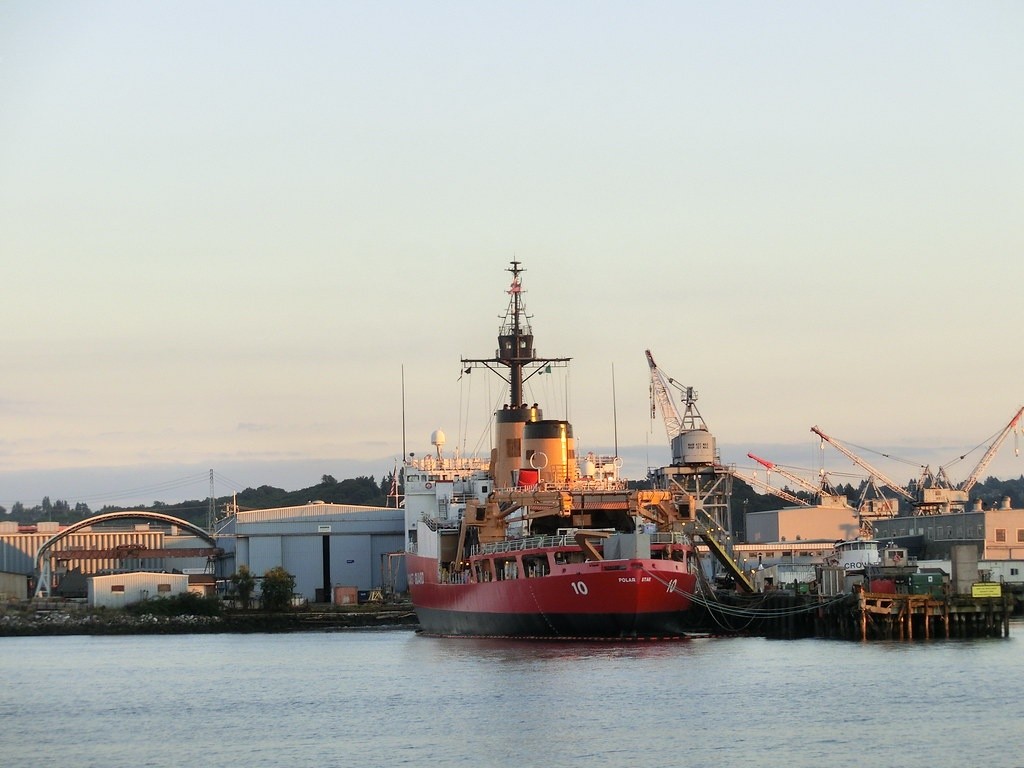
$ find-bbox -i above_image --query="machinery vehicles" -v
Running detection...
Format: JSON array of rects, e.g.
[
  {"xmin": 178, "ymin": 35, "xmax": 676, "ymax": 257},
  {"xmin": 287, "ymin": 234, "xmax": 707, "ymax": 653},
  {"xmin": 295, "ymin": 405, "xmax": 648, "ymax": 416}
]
[{"xmin": 641, "ymin": 345, "xmax": 1024, "ymax": 516}]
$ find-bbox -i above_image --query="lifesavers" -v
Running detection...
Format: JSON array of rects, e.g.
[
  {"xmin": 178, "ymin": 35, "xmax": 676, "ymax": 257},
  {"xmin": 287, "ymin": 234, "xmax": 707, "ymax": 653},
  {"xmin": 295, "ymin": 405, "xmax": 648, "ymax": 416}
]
[
  {"xmin": 893, "ymin": 555, "xmax": 901, "ymax": 562},
  {"xmin": 425, "ymin": 483, "xmax": 432, "ymax": 489}
]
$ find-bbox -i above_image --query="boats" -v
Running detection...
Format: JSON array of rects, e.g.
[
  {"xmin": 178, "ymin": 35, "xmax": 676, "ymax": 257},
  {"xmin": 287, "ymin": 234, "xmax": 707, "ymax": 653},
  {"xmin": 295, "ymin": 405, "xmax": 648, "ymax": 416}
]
[{"xmin": 397, "ymin": 260, "xmax": 696, "ymax": 646}]
